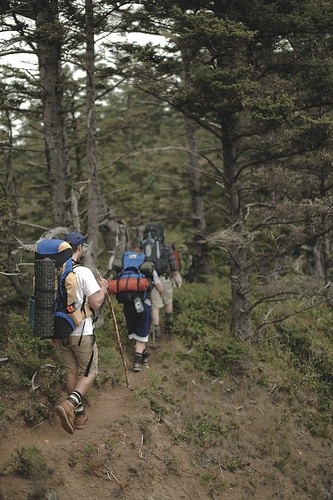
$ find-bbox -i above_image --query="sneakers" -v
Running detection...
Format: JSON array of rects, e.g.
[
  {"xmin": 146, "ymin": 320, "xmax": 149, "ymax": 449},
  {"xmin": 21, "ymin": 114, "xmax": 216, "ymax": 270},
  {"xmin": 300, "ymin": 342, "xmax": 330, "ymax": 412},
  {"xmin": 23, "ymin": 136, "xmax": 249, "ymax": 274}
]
[
  {"xmin": 75, "ymin": 415, "xmax": 88, "ymax": 430},
  {"xmin": 56, "ymin": 401, "xmax": 76, "ymax": 435}
]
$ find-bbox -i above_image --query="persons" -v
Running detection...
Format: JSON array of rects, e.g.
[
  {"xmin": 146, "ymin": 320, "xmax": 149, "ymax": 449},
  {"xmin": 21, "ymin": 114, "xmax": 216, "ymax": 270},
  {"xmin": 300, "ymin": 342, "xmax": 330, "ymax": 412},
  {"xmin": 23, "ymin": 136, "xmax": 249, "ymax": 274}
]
[
  {"xmin": 139, "ymin": 223, "xmax": 183, "ymax": 342},
  {"xmin": 113, "ymin": 240, "xmax": 163, "ymax": 371},
  {"xmin": 29, "ymin": 232, "xmax": 110, "ymax": 436}
]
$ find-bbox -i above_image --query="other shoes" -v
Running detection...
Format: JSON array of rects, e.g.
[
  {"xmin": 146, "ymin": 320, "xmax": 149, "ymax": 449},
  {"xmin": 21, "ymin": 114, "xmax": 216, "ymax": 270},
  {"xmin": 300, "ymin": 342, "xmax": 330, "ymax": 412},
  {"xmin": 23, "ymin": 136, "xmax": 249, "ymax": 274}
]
[
  {"xmin": 143, "ymin": 352, "xmax": 151, "ymax": 366},
  {"xmin": 165, "ymin": 325, "xmax": 174, "ymax": 335},
  {"xmin": 155, "ymin": 333, "xmax": 163, "ymax": 341},
  {"xmin": 133, "ymin": 361, "xmax": 142, "ymax": 371}
]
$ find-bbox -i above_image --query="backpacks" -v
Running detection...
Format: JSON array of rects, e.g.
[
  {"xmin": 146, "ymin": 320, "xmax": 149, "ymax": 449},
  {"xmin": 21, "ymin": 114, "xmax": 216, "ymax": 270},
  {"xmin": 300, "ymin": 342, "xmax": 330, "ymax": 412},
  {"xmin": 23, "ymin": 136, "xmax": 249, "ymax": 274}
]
[
  {"xmin": 106, "ymin": 252, "xmax": 155, "ymax": 307},
  {"xmin": 28, "ymin": 239, "xmax": 95, "ymax": 339},
  {"xmin": 140, "ymin": 223, "xmax": 175, "ymax": 279}
]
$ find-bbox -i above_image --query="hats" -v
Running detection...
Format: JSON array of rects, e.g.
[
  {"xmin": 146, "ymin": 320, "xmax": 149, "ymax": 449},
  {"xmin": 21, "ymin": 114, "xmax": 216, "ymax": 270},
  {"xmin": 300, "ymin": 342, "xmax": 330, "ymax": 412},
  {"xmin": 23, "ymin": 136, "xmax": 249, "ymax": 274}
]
[{"xmin": 63, "ymin": 232, "xmax": 88, "ymax": 246}]
